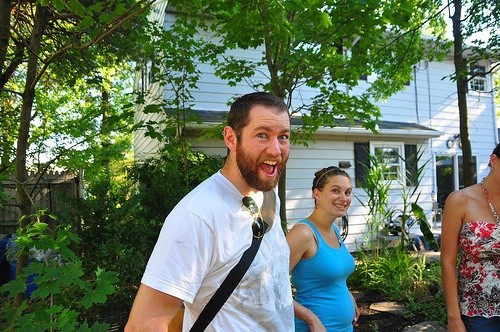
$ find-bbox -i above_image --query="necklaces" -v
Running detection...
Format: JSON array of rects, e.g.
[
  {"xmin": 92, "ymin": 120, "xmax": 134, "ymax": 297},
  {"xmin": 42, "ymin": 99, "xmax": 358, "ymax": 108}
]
[{"xmin": 481, "ymin": 176, "xmax": 500, "ymax": 224}]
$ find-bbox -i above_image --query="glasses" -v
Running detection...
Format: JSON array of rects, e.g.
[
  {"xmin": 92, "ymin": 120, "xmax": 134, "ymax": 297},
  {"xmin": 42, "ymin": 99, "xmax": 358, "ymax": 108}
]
[{"xmin": 242, "ymin": 195, "xmax": 264, "ymax": 238}]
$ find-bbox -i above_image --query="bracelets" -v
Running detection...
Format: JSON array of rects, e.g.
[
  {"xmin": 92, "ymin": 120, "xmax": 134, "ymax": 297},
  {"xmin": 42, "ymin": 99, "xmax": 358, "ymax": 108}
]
[{"xmin": 302, "ymin": 308, "xmax": 310, "ymax": 322}]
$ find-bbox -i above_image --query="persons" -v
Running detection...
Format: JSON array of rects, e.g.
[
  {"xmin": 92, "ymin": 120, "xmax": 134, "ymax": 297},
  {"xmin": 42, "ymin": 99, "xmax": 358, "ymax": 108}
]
[
  {"xmin": 440, "ymin": 142, "xmax": 500, "ymax": 332},
  {"xmin": 124, "ymin": 91, "xmax": 295, "ymax": 332},
  {"xmin": 285, "ymin": 166, "xmax": 360, "ymax": 332}
]
